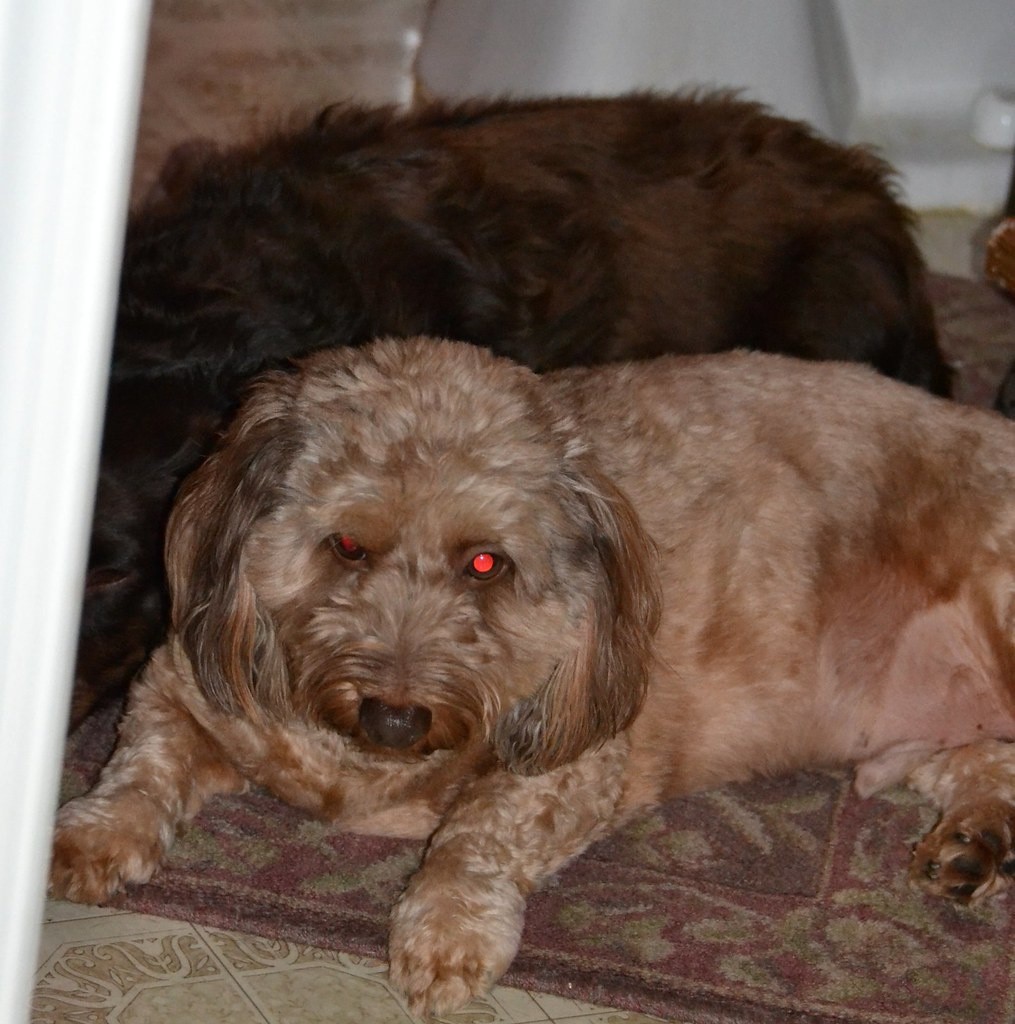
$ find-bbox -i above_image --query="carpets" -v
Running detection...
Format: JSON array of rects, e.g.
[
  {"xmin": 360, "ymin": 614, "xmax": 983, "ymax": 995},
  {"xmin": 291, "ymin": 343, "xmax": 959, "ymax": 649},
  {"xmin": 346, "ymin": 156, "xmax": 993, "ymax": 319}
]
[{"xmin": 60, "ymin": 269, "xmax": 1015, "ymax": 1024}]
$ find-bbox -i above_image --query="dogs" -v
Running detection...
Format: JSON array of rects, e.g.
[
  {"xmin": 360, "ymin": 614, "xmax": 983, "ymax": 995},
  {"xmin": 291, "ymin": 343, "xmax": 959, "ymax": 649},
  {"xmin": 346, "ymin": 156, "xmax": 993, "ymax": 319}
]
[{"xmin": 44, "ymin": 82, "xmax": 1014, "ymax": 1024}]
